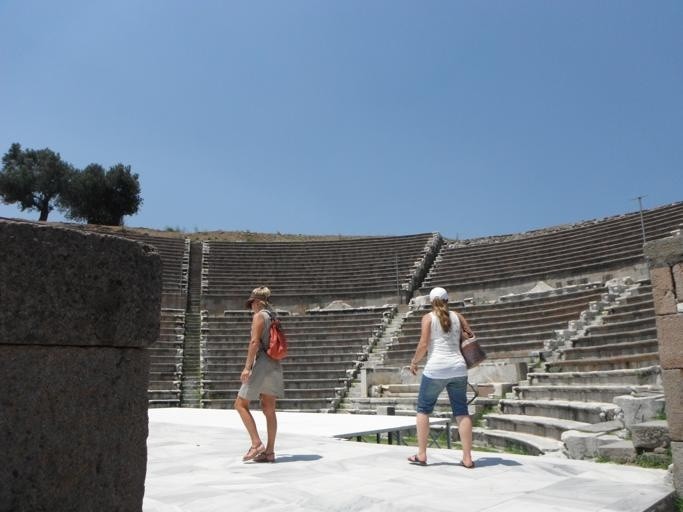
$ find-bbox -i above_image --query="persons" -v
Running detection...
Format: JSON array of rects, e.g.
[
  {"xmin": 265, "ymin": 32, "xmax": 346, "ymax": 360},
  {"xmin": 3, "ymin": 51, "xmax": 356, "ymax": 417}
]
[
  {"xmin": 232, "ymin": 284, "xmax": 286, "ymax": 465},
  {"xmin": 405, "ymin": 284, "xmax": 479, "ymax": 469}
]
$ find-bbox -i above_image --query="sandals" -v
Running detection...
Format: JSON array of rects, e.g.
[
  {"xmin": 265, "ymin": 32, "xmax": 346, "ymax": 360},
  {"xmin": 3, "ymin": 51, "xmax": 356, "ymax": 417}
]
[
  {"xmin": 242, "ymin": 442, "xmax": 265, "ymax": 462},
  {"xmin": 253, "ymin": 451, "xmax": 274, "ymax": 463}
]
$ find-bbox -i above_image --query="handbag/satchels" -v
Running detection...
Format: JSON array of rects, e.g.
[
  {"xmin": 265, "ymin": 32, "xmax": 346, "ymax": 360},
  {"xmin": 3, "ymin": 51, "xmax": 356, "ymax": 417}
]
[{"xmin": 460, "ymin": 328, "xmax": 487, "ymax": 370}]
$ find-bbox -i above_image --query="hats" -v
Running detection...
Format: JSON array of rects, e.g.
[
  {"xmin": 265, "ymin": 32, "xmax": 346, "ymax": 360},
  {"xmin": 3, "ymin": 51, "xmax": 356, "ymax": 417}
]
[
  {"xmin": 429, "ymin": 287, "xmax": 448, "ymax": 303},
  {"xmin": 245, "ymin": 286, "xmax": 271, "ymax": 302}
]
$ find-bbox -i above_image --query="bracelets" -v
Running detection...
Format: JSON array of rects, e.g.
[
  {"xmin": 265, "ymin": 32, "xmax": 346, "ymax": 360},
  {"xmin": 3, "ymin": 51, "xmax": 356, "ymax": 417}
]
[{"xmin": 411, "ymin": 361, "xmax": 416, "ymax": 366}]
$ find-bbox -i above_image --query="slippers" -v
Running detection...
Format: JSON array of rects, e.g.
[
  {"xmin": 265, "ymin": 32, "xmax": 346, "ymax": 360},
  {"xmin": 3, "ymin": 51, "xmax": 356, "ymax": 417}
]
[
  {"xmin": 459, "ymin": 458, "xmax": 475, "ymax": 468},
  {"xmin": 407, "ymin": 454, "xmax": 427, "ymax": 465}
]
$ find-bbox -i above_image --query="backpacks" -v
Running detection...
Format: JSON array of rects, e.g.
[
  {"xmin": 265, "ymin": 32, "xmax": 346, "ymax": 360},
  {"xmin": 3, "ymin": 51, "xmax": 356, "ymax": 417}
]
[{"xmin": 257, "ymin": 309, "xmax": 288, "ymax": 360}]
